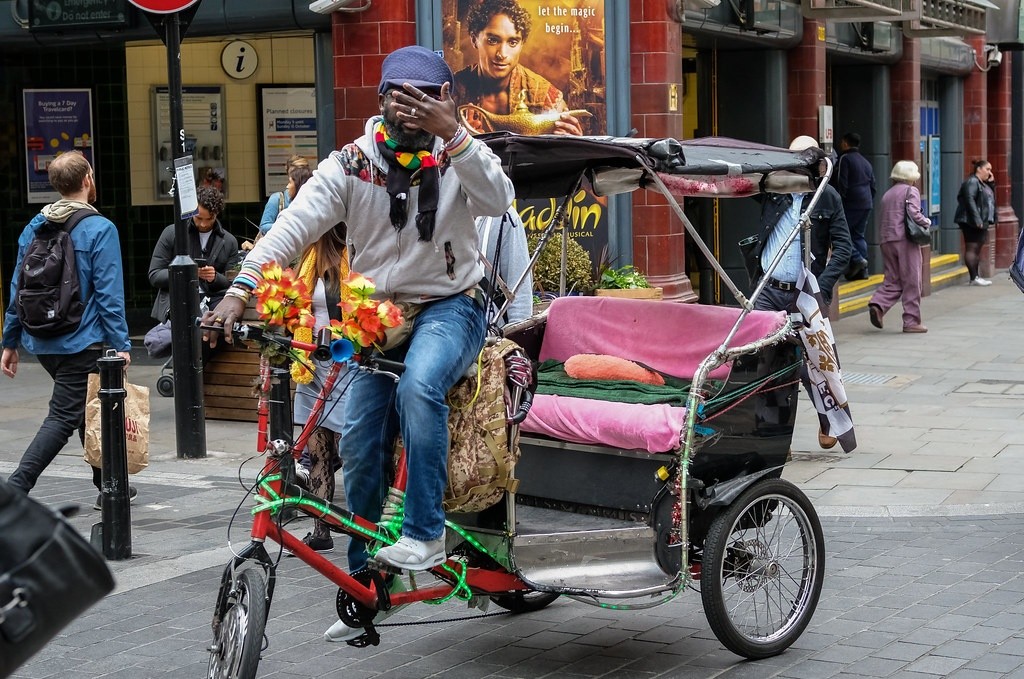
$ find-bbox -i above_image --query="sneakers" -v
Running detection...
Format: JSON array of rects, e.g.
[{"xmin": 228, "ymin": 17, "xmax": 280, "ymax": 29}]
[
  {"xmin": 374, "ymin": 527, "xmax": 447, "ymax": 571},
  {"xmin": 323, "ymin": 573, "xmax": 413, "ymax": 642}
]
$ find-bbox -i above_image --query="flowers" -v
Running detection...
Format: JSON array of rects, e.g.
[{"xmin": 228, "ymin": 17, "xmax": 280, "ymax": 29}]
[{"xmin": 251, "ymin": 262, "xmax": 409, "ymax": 385}]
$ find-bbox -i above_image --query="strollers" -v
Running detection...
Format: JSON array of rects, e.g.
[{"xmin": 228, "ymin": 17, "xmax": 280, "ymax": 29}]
[{"xmin": 145, "ymin": 247, "xmax": 249, "ymax": 398}]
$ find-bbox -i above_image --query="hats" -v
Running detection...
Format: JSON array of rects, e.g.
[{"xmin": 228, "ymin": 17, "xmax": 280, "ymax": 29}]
[{"xmin": 377, "ymin": 45, "xmax": 454, "ymax": 98}]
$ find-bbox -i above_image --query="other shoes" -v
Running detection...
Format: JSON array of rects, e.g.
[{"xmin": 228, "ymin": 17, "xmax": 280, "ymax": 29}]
[
  {"xmin": 93, "ymin": 486, "xmax": 137, "ymax": 510},
  {"xmin": 903, "ymin": 324, "xmax": 929, "ymax": 333},
  {"xmin": 970, "ymin": 276, "xmax": 992, "ymax": 286},
  {"xmin": 818, "ymin": 425, "xmax": 837, "ymax": 448},
  {"xmin": 282, "ymin": 532, "xmax": 335, "ymax": 555},
  {"xmin": 869, "ymin": 305, "xmax": 883, "ymax": 329}
]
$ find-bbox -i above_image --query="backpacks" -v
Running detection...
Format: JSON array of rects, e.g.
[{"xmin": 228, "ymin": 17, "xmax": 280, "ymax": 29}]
[{"xmin": 16, "ymin": 208, "xmax": 103, "ymax": 340}]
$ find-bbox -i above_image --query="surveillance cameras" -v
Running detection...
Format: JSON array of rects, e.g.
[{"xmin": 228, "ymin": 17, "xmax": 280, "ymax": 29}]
[
  {"xmin": 987, "ymin": 51, "xmax": 1002, "ymax": 66},
  {"xmin": 310, "ymin": 0, "xmax": 354, "ymax": 15}
]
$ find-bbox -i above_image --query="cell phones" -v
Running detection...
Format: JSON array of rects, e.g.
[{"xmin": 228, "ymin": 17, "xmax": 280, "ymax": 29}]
[
  {"xmin": 988, "ymin": 174, "xmax": 991, "ymax": 179},
  {"xmin": 194, "ymin": 258, "xmax": 207, "ymax": 268}
]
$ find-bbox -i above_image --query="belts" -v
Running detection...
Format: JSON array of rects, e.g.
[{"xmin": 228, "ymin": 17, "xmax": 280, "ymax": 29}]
[{"xmin": 760, "ymin": 271, "xmax": 804, "ymax": 292}]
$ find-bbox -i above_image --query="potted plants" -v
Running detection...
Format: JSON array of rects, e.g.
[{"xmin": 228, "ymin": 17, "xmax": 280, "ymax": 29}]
[{"xmin": 596, "ymin": 267, "xmax": 663, "ymax": 299}]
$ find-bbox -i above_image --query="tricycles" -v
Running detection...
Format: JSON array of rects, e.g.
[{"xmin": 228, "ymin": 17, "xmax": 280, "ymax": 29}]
[{"xmin": 205, "ymin": 127, "xmax": 833, "ymax": 679}]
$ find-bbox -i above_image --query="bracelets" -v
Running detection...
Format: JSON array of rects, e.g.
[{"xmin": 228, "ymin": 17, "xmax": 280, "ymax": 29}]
[{"xmin": 223, "ymin": 283, "xmax": 255, "ymax": 307}]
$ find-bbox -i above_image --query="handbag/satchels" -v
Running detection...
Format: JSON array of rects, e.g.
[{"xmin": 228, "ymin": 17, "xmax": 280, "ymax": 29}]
[
  {"xmin": 83, "ymin": 365, "xmax": 151, "ymax": 473},
  {"xmin": 0, "ymin": 475, "xmax": 117, "ymax": 679},
  {"xmin": 904, "ymin": 186, "xmax": 932, "ymax": 247},
  {"xmin": 372, "ymin": 299, "xmax": 427, "ymax": 353},
  {"xmin": 1009, "ymin": 224, "xmax": 1024, "ymax": 293},
  {"xmin": 144, "ymin": 317, "xmax": 173, "ymax": 360}
]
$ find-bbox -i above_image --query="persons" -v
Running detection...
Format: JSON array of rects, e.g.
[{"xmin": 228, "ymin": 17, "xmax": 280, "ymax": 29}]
[
  {"xmin": 953, "ymin": 159, "xmax": 997, "ymax": 286},
  {"xmin": 257, "ymin": 154, "xmax": 312, "ymax": 238},
  {"xmin": 0, "ymin": 150, "xmax": 137, "ymax": 513},
  {"xmin": 199, "ymin": 45, "xmax": 515, "ymax": 643},
  {"xmin": 144, "ymin": 184, "xmax": 249, "ymax": 373},
  {"xmin": 868, "ymin": 159, "xmax": 932, "ymax": 333},
  {"xmin": 448, "ymin": 1, "xmax": 585, "ymax": 139},
  {"xmin": 256, "ymin": 220, "xmax": 355, "ymax": 557},
  {"xmin": 830, "ymin": 131, "xmax": 877, "ymax": 282},
  {"xmin": 475, "ymin": 203, "xmax": 535, "ymax": 320},
  {"xmin": 744, "ymin": 135, "xmax": 851, "ymax": 448}
]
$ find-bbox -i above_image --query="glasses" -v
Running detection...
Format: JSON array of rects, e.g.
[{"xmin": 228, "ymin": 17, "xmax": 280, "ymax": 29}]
[{"xmin": 386, "ymin": 88, "xmax": 443, "ymax": 108}]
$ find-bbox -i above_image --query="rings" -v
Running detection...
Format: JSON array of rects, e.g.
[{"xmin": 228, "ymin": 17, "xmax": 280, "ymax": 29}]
[
  {"xmin": 411, "ymin": 108, "xmax": 419, "ymax": 117},
  {"xmin": 214, "ymin": 318, "xmax": 224, "ymax": 324},
  {"xmin": 420, "ymin": 94, "xmax": 427, "ymax": 102}
]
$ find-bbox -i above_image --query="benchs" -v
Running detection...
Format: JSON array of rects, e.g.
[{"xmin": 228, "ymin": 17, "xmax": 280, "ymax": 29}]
[{"xmin": 513, "ymin": 297, "xmax": 792, "ymax": 451}]
